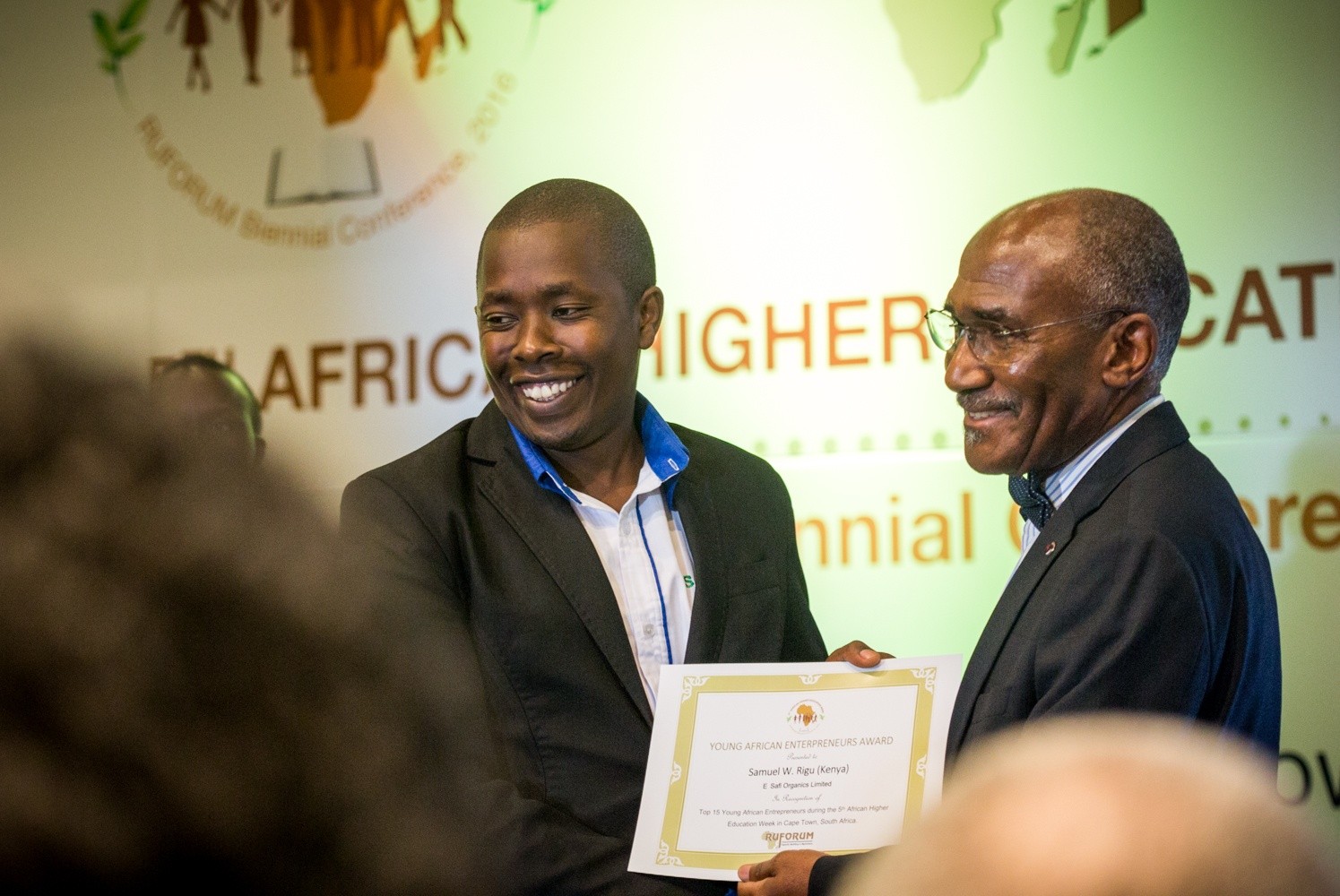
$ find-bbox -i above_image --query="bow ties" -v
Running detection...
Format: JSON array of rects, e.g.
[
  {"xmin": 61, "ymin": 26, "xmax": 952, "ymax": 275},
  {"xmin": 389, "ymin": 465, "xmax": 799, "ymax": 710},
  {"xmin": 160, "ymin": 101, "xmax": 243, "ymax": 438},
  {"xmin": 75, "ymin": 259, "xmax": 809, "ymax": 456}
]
[{"xmin": 1006, "ymin": 472, "xmax": 1058, "ymax": 532}]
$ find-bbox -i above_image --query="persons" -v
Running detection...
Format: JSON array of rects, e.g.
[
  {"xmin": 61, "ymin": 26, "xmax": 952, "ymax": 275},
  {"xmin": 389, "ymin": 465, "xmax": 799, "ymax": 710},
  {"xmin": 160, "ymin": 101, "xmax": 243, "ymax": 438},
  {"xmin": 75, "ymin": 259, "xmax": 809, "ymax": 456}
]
[
  {"xmin": 733, "ymin": 188, "xmax": 1282, "ymax": 896},
  {"xmin": 0, "ymin": 280, "xmax": 540, "ymax": 896},
  {"xmin": 338, "ymin": 180, "xmax": 901, "ymax": 896},
  {"xmin": 838, "ymin": 707, "xmax": 1340, "ymax": 896}
]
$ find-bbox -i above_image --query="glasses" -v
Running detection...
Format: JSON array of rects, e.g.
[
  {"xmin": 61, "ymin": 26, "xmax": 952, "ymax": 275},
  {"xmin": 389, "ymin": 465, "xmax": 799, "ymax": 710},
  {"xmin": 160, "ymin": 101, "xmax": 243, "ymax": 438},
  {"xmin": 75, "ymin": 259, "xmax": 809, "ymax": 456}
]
[{"xmin": 923, "ymin": 308, "xmax": 1138, "ymax": 367}]
